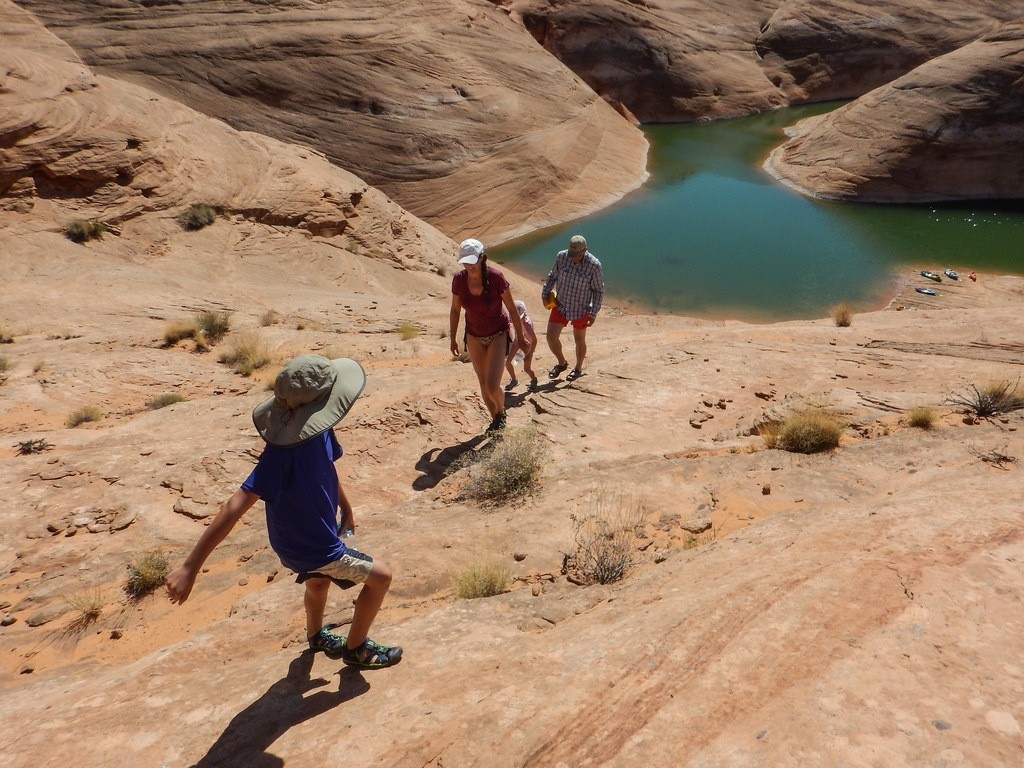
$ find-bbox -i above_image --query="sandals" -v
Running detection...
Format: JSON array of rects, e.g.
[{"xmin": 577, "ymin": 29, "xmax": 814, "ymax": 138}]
[
  {"xmin": 342, "ymin": 637, "xmax": 402, "ymax": 669},
  {"xmin": 504, "ymin": 379, "xmax": 518, "ymax": 390},
  {"xmin": 307, "ymin": 627, "xmax": 347, "ymax": 660},
  {"xmin": 566, "ymin": 369, "xmax": 581, "ymax": 380},
  {"xmin": 548, "ymin": 360, "xmax": 568, "ymax": 378},
  {"xmin": 530, "ymin": 378, "xmax": 538, "ymax": 389}
]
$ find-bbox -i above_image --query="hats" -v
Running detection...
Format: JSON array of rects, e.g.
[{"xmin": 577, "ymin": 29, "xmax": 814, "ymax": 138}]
[
  {"xmin": 513, "ymin": 300, "xmax": 526, "ymax": 319},
  {"xmin": 251, "ymin": 354, "xmax": 366, "ymax": 448},
  {"xmin": 567, "ymin": 235, "xmax": 587, "ymax": 258},
  {"xmin": 457, "ymin": 238, "xmax": 483, "ymax": 264}
]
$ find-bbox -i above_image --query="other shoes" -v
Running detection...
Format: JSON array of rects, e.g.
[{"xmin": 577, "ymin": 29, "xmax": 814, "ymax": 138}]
[{"xmin": 485, "ymin": 410, "xmax": 507, "ymax": 434}]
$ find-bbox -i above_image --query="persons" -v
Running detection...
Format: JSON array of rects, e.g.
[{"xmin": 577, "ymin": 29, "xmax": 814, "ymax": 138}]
[
  {"xmin": 504, "ymin": 300, "xmax": 538, "ymax": 391},
  {"xmin": 164, "ymin": 354, "xmax": 404, "ymax": 668},
  {"xmin": 449, "ymin": 238, "xmax": 531, "ymax": 440},
  {"xmin": 968, "ymin": 271, "xmax": 977, "ymax": 282},
  {"xmin": 541, "ymin": 235, "xmax": 605, "ymax": 382}
]
